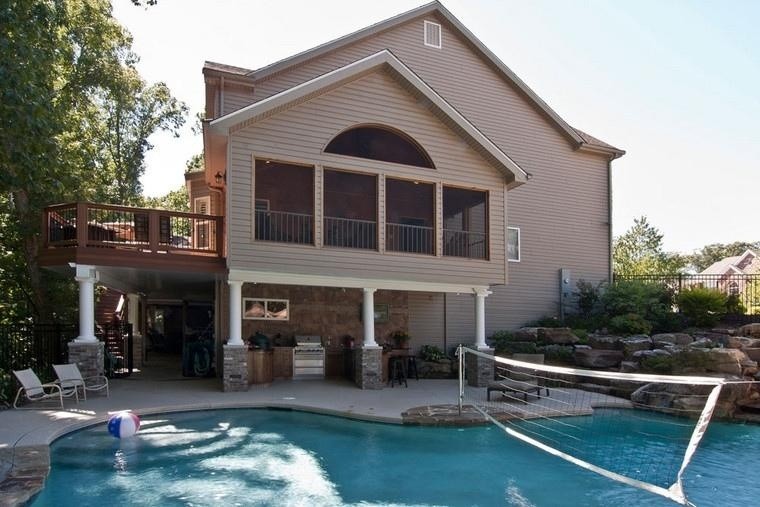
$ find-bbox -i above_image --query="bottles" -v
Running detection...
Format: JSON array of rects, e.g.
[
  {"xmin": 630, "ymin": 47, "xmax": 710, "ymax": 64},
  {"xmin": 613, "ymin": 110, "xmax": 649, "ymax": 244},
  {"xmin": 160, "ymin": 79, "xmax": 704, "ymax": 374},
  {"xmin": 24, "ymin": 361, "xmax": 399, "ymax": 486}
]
[{"xmin": 350, "ymin": 337, "xmax": 355, "ymax": 348}]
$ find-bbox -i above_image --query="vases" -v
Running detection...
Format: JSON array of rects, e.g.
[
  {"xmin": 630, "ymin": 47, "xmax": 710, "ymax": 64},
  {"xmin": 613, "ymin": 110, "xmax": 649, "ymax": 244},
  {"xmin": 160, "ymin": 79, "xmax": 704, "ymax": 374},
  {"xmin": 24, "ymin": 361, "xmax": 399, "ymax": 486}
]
[{"xmin": 399, "ymin": 338, "xmax": 405, "ymax": 349}]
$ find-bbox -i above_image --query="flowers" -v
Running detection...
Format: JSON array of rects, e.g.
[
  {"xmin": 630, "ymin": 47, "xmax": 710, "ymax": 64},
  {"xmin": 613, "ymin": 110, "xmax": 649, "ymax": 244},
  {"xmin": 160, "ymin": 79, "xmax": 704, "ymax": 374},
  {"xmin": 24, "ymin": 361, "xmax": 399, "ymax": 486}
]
[{"xmin": 390, "ymin": 329, "xmax": 413, "ymax": 343}]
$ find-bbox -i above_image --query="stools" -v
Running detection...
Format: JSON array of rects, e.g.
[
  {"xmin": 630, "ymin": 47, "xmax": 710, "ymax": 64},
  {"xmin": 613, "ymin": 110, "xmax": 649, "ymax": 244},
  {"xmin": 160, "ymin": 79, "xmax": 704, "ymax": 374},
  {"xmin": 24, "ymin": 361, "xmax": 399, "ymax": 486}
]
[{"xmin": 386, "ymin": 355, "xmax": 419, "ymax": 389}]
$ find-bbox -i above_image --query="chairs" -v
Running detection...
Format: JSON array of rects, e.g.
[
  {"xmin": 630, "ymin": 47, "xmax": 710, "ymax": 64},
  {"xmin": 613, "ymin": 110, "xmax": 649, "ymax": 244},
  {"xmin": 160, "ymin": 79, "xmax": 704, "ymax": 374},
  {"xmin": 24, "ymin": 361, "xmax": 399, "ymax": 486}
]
[
  {"xmin": 485, "ymin": 352, "xmax": 551, "ymax": 405},
  {"xmin": 10, "ymin": 361, "xmax": 111, "ymax": 411}
]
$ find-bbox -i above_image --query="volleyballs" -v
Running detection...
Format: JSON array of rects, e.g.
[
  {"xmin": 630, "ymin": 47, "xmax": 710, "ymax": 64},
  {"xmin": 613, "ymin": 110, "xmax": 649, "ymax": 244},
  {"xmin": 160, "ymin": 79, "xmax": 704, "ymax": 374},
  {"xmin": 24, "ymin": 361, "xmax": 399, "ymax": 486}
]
[{"xmin": 108, "ymin": 413, "xmax": 140, "ymax": 438}]
